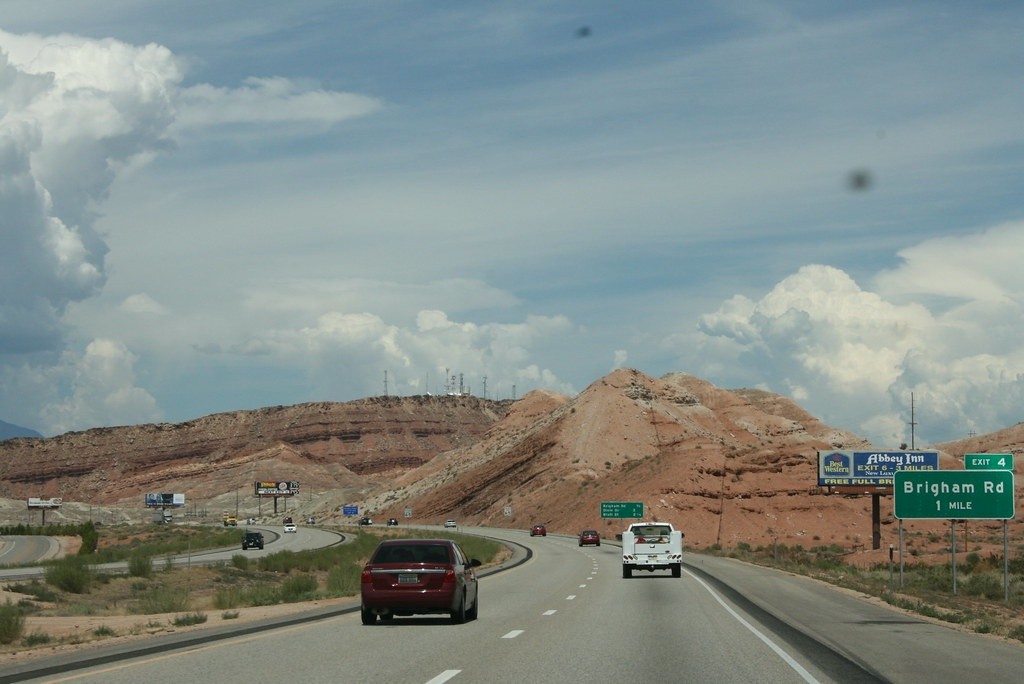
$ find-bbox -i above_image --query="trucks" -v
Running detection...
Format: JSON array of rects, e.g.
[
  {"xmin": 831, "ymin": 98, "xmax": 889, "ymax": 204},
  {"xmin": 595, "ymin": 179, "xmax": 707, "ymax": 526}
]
[
  {"xmin": 152, "ymin": 511, "xmax": 173, "ymax": 524},
  {"xmin": 28, "ymin": 498, "xmax": 62, "ymax": 508}
]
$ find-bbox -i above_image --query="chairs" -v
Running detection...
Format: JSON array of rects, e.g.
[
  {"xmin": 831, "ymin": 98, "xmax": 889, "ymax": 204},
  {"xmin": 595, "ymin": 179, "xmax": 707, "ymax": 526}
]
[
  {"xmin": 391, "ymin": 547, "xmax": 416, "ymax": 561},
  {"xmin": 634, "ymin": 528, "xmax": 642, "ymax": 535},
  {"xmin": 660, "ymin": 529, "xmax": 668, "ymax": 535}
]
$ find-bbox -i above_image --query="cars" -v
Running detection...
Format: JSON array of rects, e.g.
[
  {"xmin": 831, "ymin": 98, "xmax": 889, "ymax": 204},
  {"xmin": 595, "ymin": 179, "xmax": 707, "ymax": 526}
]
[
  {"xmin": 444, "ymin": 520, "xmax": 457, "ymax": 528},
  {"xmin": 247, "ymin": 518, "xmax": 256, "ymax": 525},
  {"xmin": 306, "ymin": 517, "xmax": 315, "ymax": 524},
  {"xmin": 283, "ymin": 517, "xmax": 292, "ymax": 524},
  {"xmin": 358, "ymin": 516, "xmax": 372, "ymax": 525},
  {"xmin": 224, "ymin": 515, "xmax": 238, "ymax": 526},
  {"xmin": 360, "ymin": 540, "xmax": 482, "ymax": 624},
  {"xmin": 283, "ymin": 523, "xmax": 297, "ymax": 533},
  {"xmin": 577, "ymin": 530, "xmax": 600, "ymax": 546},
  {"xmin": 616, "ymin": 522, "xmax": 686, "ymax": 578},
  {"xmin": 242, "ymin": 532, "xmax": 264, "ymax": 550},
  {"xmin": 530, "ymin": 524, "xmax": 546, "ymax": 536},
  {"xmin": 387, "ymin": 517, "xmax": 398, "ymax": 526}
]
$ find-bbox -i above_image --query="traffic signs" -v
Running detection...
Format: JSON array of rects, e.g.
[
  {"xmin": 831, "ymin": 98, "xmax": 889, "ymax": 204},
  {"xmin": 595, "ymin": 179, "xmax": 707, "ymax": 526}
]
[
  {"xmin": 343, "ymin": 507, "xmax": 358, "ymax": 515},
  {"xmin": 600, "ymin": 501, "xmax": 644, "ymax": 519}
]
